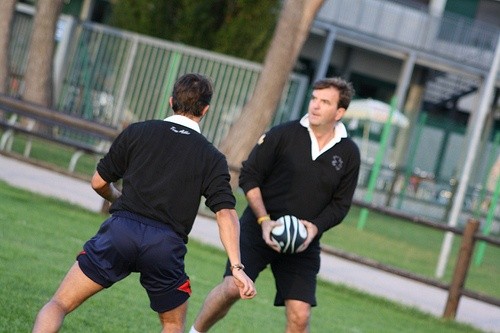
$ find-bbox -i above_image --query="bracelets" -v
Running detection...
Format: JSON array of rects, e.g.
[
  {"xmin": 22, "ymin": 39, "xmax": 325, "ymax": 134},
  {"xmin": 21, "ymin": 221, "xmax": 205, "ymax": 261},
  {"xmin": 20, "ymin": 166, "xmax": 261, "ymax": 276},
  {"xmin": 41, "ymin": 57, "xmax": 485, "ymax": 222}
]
[{"xmin": 259, "ymin": 216, "xmax": 270, "ymax": 224}]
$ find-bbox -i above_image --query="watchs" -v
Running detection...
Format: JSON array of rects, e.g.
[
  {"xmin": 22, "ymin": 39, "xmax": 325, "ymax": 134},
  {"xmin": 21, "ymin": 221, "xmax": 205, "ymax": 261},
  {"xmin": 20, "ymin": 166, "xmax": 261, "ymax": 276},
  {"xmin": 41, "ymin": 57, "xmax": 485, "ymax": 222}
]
[{"xmin": 230, "ymin": 264, "xmax": 244, "ymax": 271}]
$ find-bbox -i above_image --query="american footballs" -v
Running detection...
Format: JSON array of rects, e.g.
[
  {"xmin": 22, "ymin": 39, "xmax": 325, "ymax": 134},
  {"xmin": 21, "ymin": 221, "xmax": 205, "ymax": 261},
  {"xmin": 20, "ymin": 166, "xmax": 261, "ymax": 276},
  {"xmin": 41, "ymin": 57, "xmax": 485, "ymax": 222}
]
[{"xmin": 272, "ymin": 215, "xmax": 308, "ymax": 254}]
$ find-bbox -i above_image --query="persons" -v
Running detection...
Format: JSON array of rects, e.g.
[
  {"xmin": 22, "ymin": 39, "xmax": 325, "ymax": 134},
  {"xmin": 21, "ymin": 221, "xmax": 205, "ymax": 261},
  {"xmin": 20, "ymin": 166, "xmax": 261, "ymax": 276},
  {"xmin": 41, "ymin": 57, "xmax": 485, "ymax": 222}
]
[
  {"xmin": 32, "ymin": 74, "xmax": 257, "ymax": 332},
  {"xmin": 188, "ymin": 79, "xmax": 361, "ymax": 333}
]
